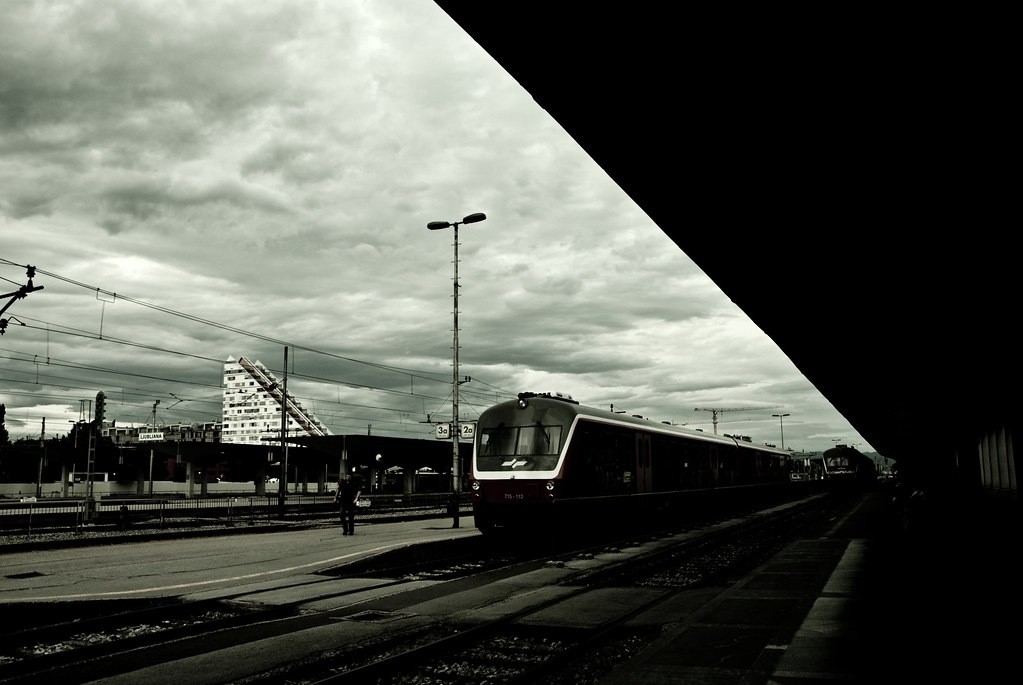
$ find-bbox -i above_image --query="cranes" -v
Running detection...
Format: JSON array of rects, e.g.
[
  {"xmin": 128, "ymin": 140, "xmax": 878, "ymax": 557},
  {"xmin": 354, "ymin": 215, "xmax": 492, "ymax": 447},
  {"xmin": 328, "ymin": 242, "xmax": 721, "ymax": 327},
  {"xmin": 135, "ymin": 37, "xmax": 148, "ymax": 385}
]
[{"xmin": 693, "ymin": 405, "xmax": 785, "ymax": 436}]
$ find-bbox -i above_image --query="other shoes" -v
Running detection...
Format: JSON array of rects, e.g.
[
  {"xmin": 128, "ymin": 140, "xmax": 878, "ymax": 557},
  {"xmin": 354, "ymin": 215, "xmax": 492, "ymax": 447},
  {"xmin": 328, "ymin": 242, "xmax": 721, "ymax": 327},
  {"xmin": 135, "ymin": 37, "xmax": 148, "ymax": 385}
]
[
  {"xmin": 349, "ymin": 531, "xmax": 353, "ymax": 535},
  {"xmin": 343, "ymin": 530, "xmax": 348, "ymax": 535}
]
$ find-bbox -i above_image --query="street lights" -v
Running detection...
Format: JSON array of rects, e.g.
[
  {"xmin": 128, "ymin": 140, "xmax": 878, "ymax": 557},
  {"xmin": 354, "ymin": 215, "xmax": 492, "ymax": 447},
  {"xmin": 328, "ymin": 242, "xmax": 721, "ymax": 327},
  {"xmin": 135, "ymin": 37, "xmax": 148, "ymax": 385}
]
[
  {"xmin": 831, "ymin": 439, "xmax": 841, "ymax": 445},
  {"xmin": 772, "ymin": 414, "xmax": 790, "ymax": 449},
  {"xmin": 852, "ymin": 443, "xmax": 862, "ymax": 450},
  {"xmin": 427, "ymin": 212, "xmax": 487, "ymax": 528},
  {"xmin": 69, "ymin": 420, "xmax": 86, "ymax": 497}
]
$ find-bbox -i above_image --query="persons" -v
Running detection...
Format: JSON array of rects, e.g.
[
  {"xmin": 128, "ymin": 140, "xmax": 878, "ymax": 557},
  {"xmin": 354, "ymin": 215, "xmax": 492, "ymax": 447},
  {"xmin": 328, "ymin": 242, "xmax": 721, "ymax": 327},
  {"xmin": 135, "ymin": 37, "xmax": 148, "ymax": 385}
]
[{"xmin": 333, "ymin": 473, "xmax": 362, "ymax": 535}]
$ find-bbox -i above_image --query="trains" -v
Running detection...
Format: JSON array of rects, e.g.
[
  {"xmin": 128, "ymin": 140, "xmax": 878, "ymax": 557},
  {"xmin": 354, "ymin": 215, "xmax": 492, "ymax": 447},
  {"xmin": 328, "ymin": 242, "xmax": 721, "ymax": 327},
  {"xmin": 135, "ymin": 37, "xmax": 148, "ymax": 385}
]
[{"xmin": 468, "ymin": 391, "xmax": 879, "ymax": 550}]
